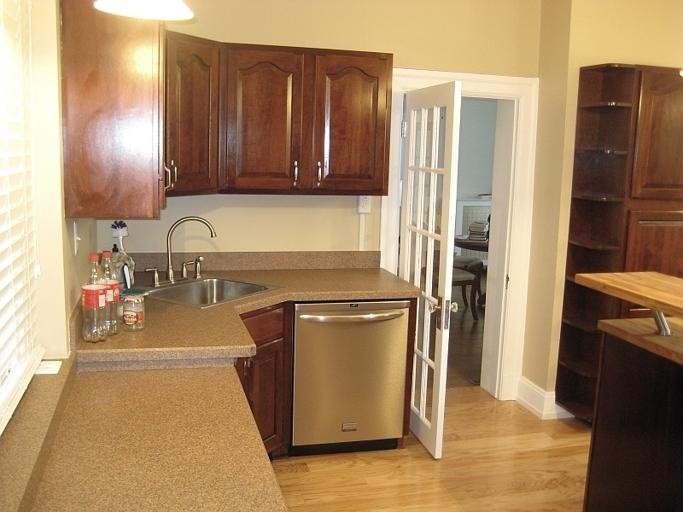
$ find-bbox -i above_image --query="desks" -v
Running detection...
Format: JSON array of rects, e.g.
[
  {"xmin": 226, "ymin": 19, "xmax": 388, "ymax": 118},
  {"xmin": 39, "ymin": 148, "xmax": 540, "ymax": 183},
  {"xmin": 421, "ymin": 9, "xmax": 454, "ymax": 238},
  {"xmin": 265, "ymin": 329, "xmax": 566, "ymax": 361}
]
[{"xmin": 573, "ymin": 271, "xmax": 683, "ymax": 512}]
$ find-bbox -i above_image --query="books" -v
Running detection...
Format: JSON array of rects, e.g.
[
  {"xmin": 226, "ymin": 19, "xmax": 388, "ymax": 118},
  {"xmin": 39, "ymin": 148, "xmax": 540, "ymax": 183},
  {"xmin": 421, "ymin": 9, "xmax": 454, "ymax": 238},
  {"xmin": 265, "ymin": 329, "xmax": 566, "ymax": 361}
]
[{"xmin": 468, "ymin": 221, "xmax": 489, "ymax": 242}]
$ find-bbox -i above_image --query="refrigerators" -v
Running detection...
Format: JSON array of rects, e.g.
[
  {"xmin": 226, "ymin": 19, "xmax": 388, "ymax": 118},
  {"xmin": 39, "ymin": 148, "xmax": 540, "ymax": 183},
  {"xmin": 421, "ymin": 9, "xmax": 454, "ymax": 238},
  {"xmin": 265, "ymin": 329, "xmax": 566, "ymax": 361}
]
[{"xmin": 288, "ymin": 300, "xmax": 411, "ymax": 457}]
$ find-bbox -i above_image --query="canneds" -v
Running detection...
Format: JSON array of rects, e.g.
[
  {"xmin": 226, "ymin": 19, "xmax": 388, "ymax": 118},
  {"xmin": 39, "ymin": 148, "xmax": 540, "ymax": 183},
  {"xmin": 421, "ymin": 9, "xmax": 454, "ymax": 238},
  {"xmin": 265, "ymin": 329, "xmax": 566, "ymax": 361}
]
[{"xmin": 121, "ymin": 293, "xmax": 146, "ymax": 330}]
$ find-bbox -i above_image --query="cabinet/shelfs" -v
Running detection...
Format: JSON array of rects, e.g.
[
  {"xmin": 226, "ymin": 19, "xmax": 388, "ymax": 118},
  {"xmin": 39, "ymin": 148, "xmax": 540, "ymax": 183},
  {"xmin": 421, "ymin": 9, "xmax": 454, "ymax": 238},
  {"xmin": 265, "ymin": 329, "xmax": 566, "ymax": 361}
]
[
  {"xmin": 555, "ymin": 64, "xmax": 683, "ymax": 430},
  {"xmin": 161, "ymin": 28, "xmax": 217, "ymax": 197},
  {"xmin": 61, "ymin": 3, "xmax": 166, "ymax": 220},
  {"xmin": 219, "ymin": 42, "xmax": 392, "ymax": 197},
  {"xmin": 235, "ymin": 302, "xmax": 293, "ymax": 457}
]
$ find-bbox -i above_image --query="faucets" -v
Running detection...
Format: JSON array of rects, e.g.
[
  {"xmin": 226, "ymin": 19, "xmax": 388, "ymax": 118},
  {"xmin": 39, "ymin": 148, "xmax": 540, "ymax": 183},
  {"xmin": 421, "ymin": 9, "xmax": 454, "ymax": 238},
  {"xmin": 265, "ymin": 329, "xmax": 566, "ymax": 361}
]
[{"xmin": 165, "ymin": 213, "xmax": 218, "ymax": 283}]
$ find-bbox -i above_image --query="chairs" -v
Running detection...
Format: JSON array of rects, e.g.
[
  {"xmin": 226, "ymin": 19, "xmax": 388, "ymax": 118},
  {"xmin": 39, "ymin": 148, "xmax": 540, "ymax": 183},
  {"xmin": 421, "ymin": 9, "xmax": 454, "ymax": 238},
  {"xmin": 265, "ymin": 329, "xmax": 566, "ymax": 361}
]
[
  {"xmin": 454, "ymin": 255, "xmax": 483, "ymax": 299},
  {"xmin": 451, "ymin": 267, "xmax": 478, "ymax": 321}
]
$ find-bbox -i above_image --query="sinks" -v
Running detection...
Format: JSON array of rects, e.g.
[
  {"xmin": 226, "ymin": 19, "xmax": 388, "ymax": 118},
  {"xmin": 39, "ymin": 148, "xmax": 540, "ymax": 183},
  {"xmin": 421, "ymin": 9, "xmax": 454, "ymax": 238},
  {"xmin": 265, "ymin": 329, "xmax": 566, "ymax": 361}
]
[{"xmin": 142, "ymin": 274, "xmax": 282, "ymax": 308}]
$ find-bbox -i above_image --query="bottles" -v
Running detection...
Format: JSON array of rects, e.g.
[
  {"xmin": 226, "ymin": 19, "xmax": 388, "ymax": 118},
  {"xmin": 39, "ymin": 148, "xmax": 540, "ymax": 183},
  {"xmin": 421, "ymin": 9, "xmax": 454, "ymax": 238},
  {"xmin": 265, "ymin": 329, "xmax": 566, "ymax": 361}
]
[{"xmin": 81, "ymin": 250, "xmax": 120, "ymax": 343}]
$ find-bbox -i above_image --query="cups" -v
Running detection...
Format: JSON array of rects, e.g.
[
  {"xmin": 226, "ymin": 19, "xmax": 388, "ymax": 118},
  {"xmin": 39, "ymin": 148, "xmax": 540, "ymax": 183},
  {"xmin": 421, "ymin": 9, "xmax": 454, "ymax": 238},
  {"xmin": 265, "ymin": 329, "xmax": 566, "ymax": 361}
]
[{"xmin": 123, "ymin": 294, "xmax": 145, "ymax": 331}]
